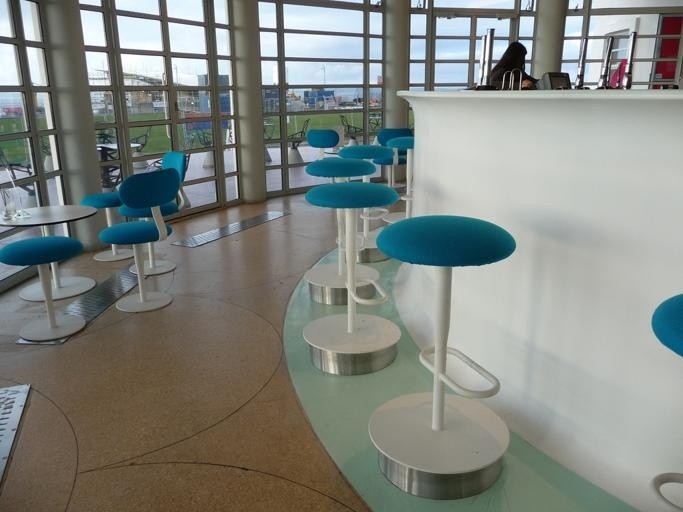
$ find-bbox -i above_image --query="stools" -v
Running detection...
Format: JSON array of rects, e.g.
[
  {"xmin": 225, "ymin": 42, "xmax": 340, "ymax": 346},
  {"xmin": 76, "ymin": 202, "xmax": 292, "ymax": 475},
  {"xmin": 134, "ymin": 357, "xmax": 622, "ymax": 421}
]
[
  {"xmin": 80, "ymin": 191, "xmax": 135, "ymax": 262},
  {"xmin": 0, "ymin": 235, "xmax": 87, "ymax": 342},
  {"xmin": 307, "ymin": 157, "xmax": 381, "ymax": 304},
  {"xmin": 367, "ymin": 215, "xmax": 519, "ymax": 502},
  {"xmin": 304, "ymin": 126, "xmax": 414, "ymax": 263},
  {"xmin": 306, "ymin": 181, "xmax": 402, "ymax": 376}
]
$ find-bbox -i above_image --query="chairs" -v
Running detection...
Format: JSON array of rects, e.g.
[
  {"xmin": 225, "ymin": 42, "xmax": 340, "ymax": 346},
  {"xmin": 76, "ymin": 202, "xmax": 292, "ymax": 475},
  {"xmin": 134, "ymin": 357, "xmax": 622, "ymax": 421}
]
[
  {"xmin": 264, "ymin": 124, "xmax": 275, "ymax": 163},
  {"xmin": 129, "ymin": 126, "xmax": 152, "ymax": 168},
  {"xmin": 339, "ymin": 112, "xmax": 384, "ymax": 147},
  {"xmin": 98, "ymin": 150, "xmax": 187, "ymax": 313},
  {"xmin": 196, "ymin": 127, "xmax": 215, "ymax": 167},
  {"xmin": 286, "ymin": 118, "xmax": 312, "ymax": 164}
]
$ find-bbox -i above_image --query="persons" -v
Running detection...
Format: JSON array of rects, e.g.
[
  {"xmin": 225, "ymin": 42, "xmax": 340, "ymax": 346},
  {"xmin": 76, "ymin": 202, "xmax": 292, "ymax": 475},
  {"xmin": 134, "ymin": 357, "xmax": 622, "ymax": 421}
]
[{"xmin": 489, "ymin": 40, "xmax": 540, "ymax": 89}]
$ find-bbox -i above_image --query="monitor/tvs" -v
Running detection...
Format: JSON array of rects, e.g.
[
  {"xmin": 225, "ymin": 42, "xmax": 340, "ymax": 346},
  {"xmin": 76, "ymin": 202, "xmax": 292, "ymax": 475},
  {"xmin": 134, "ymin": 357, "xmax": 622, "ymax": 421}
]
[{"xmin": 536, "ymin": 71, "xmax": 571, "ymax": 90}]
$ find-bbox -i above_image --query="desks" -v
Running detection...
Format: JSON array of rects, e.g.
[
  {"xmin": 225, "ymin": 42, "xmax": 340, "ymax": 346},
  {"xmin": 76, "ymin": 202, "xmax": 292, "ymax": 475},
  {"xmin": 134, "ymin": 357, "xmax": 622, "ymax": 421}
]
[{"xmin": 0, "ymin": 205, "xmax": 97, "ymax": 302}]
